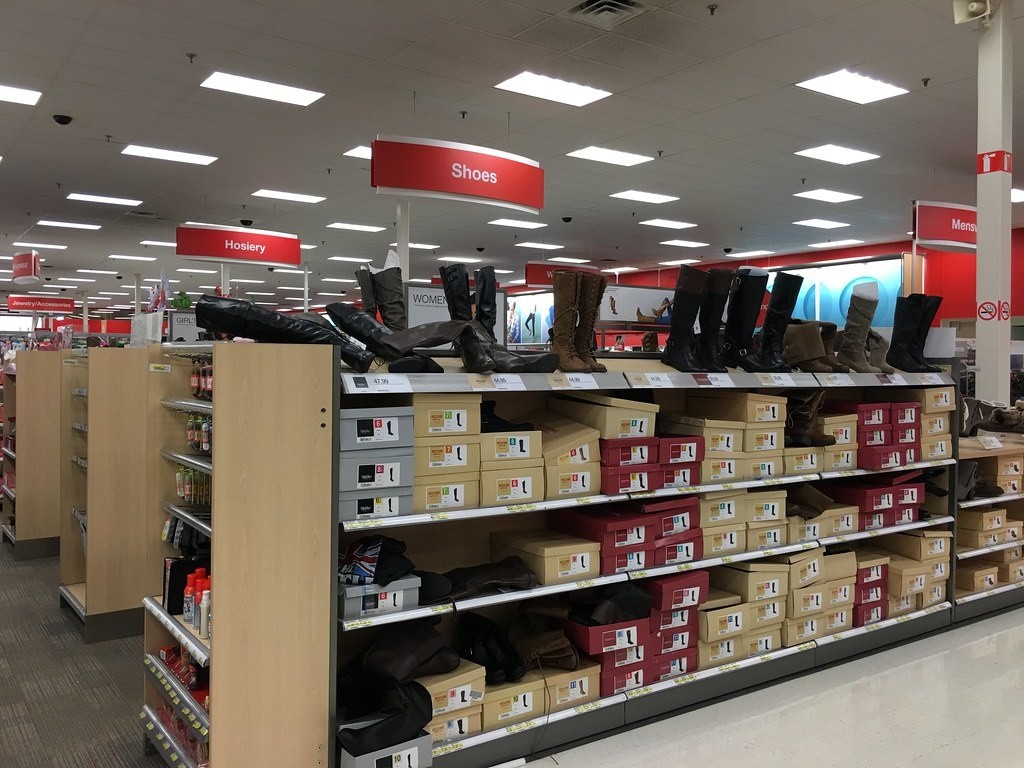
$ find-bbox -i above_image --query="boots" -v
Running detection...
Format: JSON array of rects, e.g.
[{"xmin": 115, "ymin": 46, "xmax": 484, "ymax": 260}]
[
  {"xmin": 660, "ymin": 264, "xmax": 735, "ymax": 373},
  {"xmin": 195, "ymin": 263, "xmax": 560, "ymax": 372},
  {"xmin": 612, "ymin": 388, "xmax": 662, "ymax": 438},
  {"xmin": 550, "ymin": 268, "xmax": 609, "ymax": 373},
  {"xmin": 336, "ymin": 535, "xmax": 536, "ymax": 619},
  {"xmin": 774, "ymin": 467, "xmax": 949, "ymax": 520},
  {"xmin": 886, "ymin": 293, "xmax": 943, "ymax": 373},
  {"xmin": 480, "ymin": 400, "xmax": 534, "ymax": 433},
  {"xmin": 610, "ymin": 296, "xmax": 667, "ymax": 323},
  {"xmin": 823, "ymin": 542, "xmax": 849, "ymax": 556},
  {"xmin": 720, "ymin": 264, "xmax": 895, "ymax": 374},
  {"xmin": 336, "ymin": 582, "xmax": 654, "ymax": 757},
  {"xmin": 960, "ymin": 397, "xmax": 1024, "ymax": 437},
  {"xmin": 957, "ymin": 459, "xmax": 1004, "ymax": 501},
  {"xmin": 779, "ymin": 387, "xmax": 836, "ymax": 448}
]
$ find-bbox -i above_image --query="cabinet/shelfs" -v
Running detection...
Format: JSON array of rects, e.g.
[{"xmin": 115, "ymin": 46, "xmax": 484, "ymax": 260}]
[
  {"xmin": 138, "ymin": 343, "xmax": 1024, "ymax": 768},
  {"xmin": 58, "ymin": 345, "xmax": 147, "ymax": 645},
  {"xmin": 0, "ymin": 349, "xmax": 60, "ymax": 562}
]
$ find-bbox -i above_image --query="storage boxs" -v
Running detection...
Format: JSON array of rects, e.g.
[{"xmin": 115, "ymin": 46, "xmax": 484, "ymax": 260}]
[
  {"xmin": 1004, "ymin": 518, "xmax": 1023, "ymax": 543},
  {"xmin": 330, "ymin": 381, "xmax": 954, "ymax": 768},
  {"xmin": 955, "ymin": 528, "xmax": 1005, "ymax": 549},
  {"xmin": 971, "ymin": 545, "xmax": 1022, "ymax": 564},
  {"xmin": 966, "ymin": 557, "xmax": 1024, "ymax": 584},
  {"xmin": 955, "ymin": 562, "xmax": 998, "ymax": 592},
  {"xmin": 981, "ymin": 475, "xmax": 1022, "ymax": 496},
  {"xmin": 978, "ymin": 454, "xmax": 1024, "ymax": 475},
  {"xmin": 956, "ymin": 506, "xmax": 1007, "ymax": 532}
]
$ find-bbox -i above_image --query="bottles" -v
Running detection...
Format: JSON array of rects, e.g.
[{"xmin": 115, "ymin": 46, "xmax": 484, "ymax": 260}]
[{"xmin": 182, "ymin": 568, "xmax": 211, "ymax": 641}]
